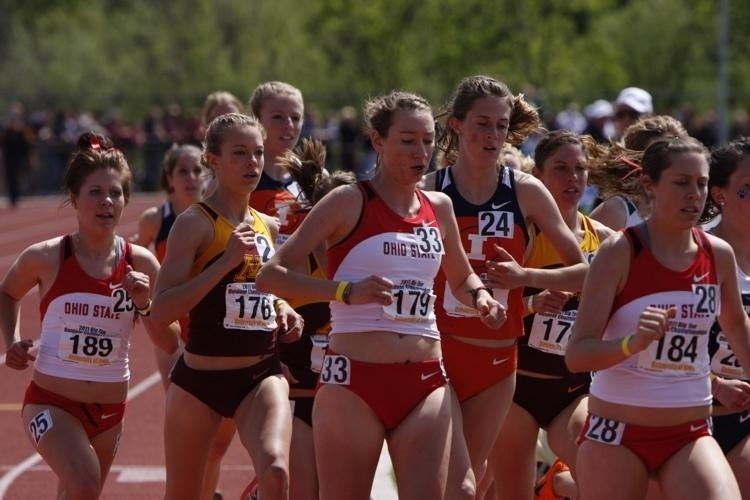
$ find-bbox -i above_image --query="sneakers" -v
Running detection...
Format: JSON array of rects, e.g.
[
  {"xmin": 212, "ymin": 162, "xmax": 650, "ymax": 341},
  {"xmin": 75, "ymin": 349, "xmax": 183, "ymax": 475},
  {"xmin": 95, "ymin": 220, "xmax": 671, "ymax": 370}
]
[{"xmin": 533, "ymin": 457, "xmax": 572, "ymax": 500}]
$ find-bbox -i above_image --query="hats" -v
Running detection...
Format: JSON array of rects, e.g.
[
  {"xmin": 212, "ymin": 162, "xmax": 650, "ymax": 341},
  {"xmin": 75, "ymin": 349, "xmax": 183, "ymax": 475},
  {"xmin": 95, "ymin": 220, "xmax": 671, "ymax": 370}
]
[{"xmin": 614, "ymin": 81, "xmax": 653, "ymax": 116}]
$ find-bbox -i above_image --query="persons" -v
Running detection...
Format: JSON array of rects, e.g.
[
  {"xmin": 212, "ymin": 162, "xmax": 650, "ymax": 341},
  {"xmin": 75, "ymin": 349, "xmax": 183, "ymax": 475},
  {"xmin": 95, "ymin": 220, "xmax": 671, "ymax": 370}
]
[{"xmin": 2, "ymin": 76, "xmax": 750, "ymax": 500}]
